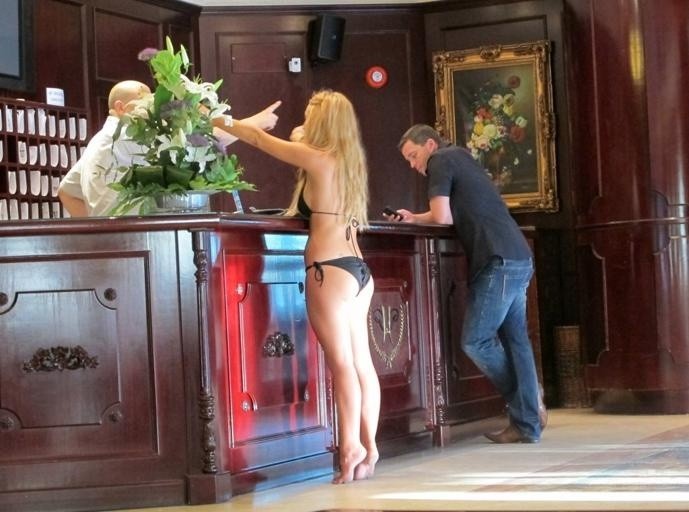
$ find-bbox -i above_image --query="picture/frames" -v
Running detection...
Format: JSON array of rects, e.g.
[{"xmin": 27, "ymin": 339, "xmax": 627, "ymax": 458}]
[{"xmin": 431, "ymin": 37, "xmax": 561, "ymax": 215}]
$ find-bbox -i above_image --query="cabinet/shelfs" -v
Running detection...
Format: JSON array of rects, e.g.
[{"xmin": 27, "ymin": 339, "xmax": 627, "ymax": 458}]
[{"xmin": 0, "ymin": 97, "xmax": 90, "ymax": 221}]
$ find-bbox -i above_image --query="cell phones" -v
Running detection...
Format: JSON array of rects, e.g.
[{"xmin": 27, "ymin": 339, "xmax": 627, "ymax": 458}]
[{"xmin": 383, "ymin": 206, "xmax": 403, "ymax": 221}]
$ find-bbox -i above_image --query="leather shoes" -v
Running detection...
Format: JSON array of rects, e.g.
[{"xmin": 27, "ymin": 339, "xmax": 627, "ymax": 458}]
[
  {"xmin": 484, "ymin": 426, "xmax": 540, "ymax": 443},
  {"xmin": 537, "ymin": 382, "xmax": 547, "ymax": 430}
]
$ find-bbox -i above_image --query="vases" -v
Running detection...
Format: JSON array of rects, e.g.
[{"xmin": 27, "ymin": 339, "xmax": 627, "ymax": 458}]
[
  {"xmin": 161, "ymin": 190, "xmax": 211, "ymax": 211},
  {"xmin": 483, "ymin": 139, "xmax": 507, "ymax": 180}
]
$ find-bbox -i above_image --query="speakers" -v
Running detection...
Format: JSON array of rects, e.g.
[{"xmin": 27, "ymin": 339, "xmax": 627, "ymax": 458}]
[{"xmin": 308, "ymin": 14, "xmax": 347, "ymax": 63}]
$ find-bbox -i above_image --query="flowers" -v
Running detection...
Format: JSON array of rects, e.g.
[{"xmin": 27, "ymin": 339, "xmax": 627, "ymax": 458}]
[
  {"xmin": 462, "ymin": 73, "xmax": 528, "ymax": 193},
  {"xmin": 102, "ymin": 33, "xmax": 257, "ymax": 219}
]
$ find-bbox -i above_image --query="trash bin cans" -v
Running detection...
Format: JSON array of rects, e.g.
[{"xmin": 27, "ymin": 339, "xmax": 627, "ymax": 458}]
[{"xmin": 550, "ymin": 323, "xmax": 593, "ymax": 409}]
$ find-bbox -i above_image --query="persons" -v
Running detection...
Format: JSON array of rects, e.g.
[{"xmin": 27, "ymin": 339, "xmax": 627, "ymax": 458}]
[
  {"xmin": 196, "ymin": 88, "xmax": 381, "ymax": 484},
  {"xmin": 58, "ymin": 80, "xmax": 283, "ymax": 218},
  {"xmin": 383, "ymin": 124, "xmax": 547, "ymax": 444}
]
